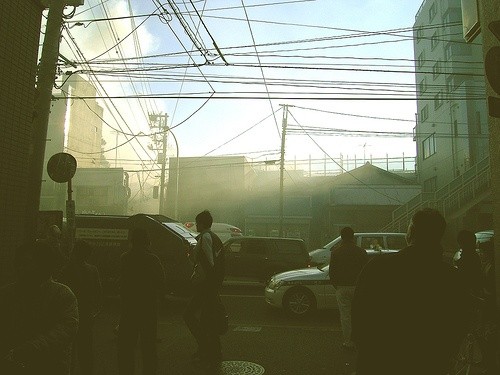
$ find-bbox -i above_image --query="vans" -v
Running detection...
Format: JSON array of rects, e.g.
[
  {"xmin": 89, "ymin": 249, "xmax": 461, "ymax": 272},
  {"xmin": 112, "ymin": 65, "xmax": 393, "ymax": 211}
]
[{"xmin": 221, "ymin": 233, "xmax": 408, "ymax": 280}]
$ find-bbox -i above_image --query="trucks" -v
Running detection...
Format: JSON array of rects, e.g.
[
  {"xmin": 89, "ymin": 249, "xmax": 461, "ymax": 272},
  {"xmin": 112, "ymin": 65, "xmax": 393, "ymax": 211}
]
[{"xmin": 69, "ymin": 213, "xmax": 200, "ymax": 278}]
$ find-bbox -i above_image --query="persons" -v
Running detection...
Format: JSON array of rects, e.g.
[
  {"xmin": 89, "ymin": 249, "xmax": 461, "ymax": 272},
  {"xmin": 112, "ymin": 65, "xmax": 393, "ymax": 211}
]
[
  {"xmin": 183, "ymin": 209, "xmax": 226, "ymax": 359},
  {"xmin": 351, "ymin": 208, "xmax": 495, "ymax": 375},
  {"xmin": 328, "ymin": 226, "xmax": 374, "ymax": 350},
  {"xmin": 0, "ymin": 223, "xmax": 168, "ymax": 375}
]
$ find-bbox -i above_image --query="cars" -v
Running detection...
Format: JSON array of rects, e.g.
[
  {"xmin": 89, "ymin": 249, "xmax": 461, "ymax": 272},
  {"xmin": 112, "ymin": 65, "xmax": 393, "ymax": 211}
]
[{"xmin": 266, "ymin": 250, "xmax": 408, "ymax": 319}]
